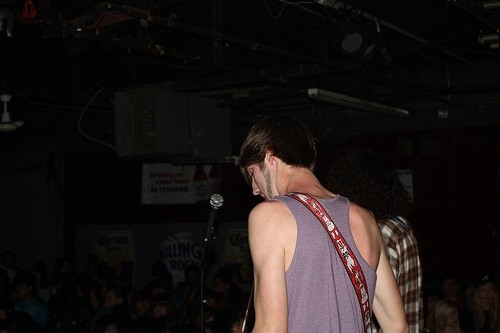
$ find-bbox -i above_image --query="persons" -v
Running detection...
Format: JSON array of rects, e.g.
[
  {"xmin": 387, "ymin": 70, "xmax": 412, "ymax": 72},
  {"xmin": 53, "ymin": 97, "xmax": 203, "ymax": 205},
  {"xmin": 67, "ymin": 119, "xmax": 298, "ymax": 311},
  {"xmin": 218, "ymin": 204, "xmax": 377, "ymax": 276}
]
[
  {"xmin": 1, "ymin": 249, "xmax": 256, "ymax": 333},
  {"xmin": 328, "ymin": 150, "xmax": 424, "ymax": 333},
  {"xmin": 426, "ymin": 275, "xmax": 499, "ymax": 332},
  {"xmin": 238, "ymin": 113, "xmax": 406, "ymax": 333}
]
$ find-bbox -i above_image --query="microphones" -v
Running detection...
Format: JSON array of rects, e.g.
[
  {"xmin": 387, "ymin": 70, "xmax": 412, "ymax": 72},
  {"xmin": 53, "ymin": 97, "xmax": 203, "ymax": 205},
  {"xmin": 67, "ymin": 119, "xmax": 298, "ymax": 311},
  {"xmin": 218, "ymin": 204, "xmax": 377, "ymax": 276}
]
[{"xmin": 204, "ymin": 194, "xmax": 224, "ymax": 243}]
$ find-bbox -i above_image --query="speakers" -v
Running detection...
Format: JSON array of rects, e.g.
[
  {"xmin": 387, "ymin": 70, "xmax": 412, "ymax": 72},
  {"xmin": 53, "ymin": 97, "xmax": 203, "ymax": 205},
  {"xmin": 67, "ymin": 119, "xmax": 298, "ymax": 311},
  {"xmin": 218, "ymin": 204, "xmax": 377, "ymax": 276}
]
[{"xmin": 113, "ymin": 87, "xmax": 232, "ymax": 160}]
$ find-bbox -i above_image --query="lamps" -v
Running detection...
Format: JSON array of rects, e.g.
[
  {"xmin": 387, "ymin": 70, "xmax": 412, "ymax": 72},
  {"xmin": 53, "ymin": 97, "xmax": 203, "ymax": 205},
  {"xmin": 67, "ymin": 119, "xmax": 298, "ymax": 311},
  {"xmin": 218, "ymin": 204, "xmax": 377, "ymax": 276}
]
[
  {"xmin": 0, "ymin": 93, "xmax": 17, "ymax": 131},
  {"xmin": 308, "ymin": 87, "xmax": 410, "ymax": 118}
]
